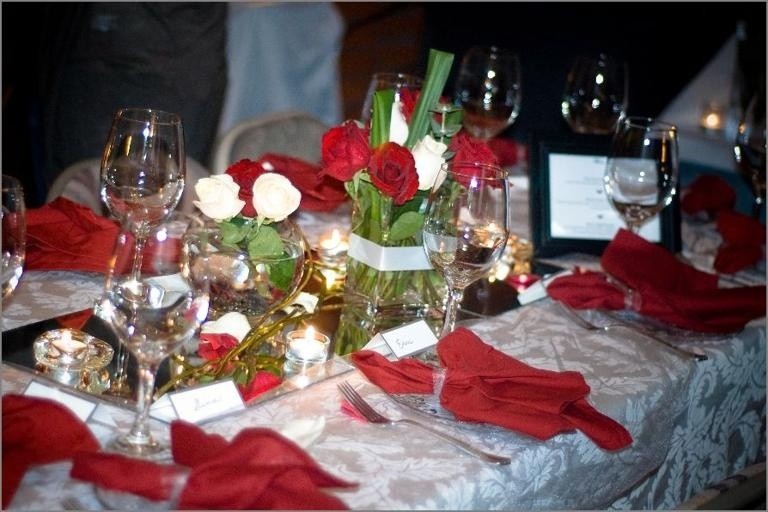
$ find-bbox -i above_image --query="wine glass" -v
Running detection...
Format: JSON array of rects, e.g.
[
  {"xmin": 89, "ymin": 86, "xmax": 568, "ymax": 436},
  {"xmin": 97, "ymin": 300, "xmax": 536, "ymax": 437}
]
[
  {"xmin": 93, "ymin": 205, "xmax": 213, "ymax": 466},
  {"xmin": 601, "ymin": 115, "xmax": 681, "ymax": 236},
  {"xmin": 407, "ymin": 160, "xmax": 513, "ymax": 369},
  {"xmin": 732, "ymin": 92, "xmax": 766, "ymax": 223},
  {"xmin": 99, "ymin": 105, "xmax": 187, "ymax": 312}
]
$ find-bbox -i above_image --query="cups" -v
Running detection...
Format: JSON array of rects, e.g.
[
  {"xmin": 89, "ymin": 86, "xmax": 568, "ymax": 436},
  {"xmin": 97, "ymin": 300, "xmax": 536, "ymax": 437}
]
[
  {"xmin": 1, "ymin": 175, "xmax": 30, "ymax": 309},
  {"xmin": 179, "ymin": 225, "xmax": 311, "ymax": 322},
  {"xmin": 358, "ymin": 73, "xmax": 426, "ymax": 125},
  {"xmin": 318, "ymin": 227, "xmax": 350, "ymax": 281},
  {"xmin": 559, "ymin": 45, "xmax": 631, "ymax": 138},
  {"xmin": 449, "ymin": 43, "xmax": 524, "ymax": 141}
]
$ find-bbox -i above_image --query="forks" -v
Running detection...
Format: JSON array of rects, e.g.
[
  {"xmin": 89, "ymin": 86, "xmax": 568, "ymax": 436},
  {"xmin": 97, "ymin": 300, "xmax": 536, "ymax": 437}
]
[{"xmin": 335, "ymin": 379, "xmax": 514, "ymax": 467}]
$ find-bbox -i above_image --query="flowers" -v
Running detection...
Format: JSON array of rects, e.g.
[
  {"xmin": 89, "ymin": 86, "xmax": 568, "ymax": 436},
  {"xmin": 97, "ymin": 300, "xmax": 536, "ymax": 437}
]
[
  {"xmin": 190, "ymin": 160, "xmax": 313, "ymax": 388},
  {"xmin": 315, "ymin": 45, "xmax": 500, "ymax": 354}
]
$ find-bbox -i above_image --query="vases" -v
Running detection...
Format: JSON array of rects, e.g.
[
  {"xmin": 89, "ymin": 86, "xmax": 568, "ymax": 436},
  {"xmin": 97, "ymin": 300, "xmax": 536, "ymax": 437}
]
[
  {"xmin": 325, "ymin": 182, "xmax": 454, "ymax": 357},
  {"xmin": 168, "ymin": 212, "xmax": 304, "ymax": 393}
]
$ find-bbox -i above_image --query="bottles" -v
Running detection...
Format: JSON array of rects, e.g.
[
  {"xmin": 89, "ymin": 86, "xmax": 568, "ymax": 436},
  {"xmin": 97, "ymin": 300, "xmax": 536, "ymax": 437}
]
[{"xmin": 554, "ymin": 297, "xmax": 710, "ymax": 364}]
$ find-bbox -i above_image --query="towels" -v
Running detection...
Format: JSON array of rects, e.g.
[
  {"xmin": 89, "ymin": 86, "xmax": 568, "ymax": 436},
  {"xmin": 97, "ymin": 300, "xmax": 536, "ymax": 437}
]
[
  {"xmin": 349, "ymin": 327, "xmax": 633, "ymax": 451},
  {"xmin": 2, "ymin": 394, "xmax": 100, "ymax": 510},
  {"xmin": 545, "ymin": 226, "xmax": 768, "ymax": 334},
  {"xmin": 676, "ymin": 171, "xmax": 767, "ymax": 273},
  {"xmin": 261, "ymin": 152, "xmax": 346, "ymax": 213},
  {"xmin": 70, "ymin": 419, "xmax": 359, "ymax": 510},
  {"xmin": 3, "ymin": 193, "xmax": 181, "ymax": 277}
]
